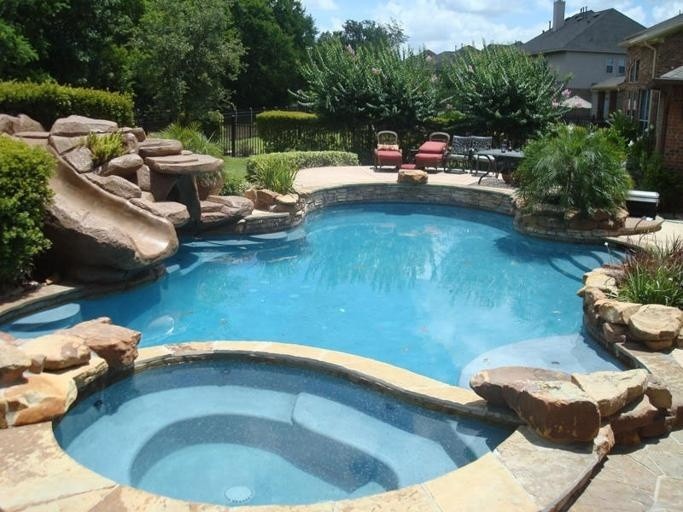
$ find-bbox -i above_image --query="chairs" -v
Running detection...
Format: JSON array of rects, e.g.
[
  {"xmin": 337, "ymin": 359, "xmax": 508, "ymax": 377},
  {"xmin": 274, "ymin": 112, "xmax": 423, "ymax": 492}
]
[{"xmin": 373, "ymin": 130, "xmax": 499, "ymax": 179}]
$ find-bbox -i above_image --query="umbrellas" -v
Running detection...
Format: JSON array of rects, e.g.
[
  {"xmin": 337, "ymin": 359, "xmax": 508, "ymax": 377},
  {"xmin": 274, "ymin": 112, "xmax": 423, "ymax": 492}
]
[{"xmin": 559, "ymin": 94, "xmax": 593, "ymax": 120}]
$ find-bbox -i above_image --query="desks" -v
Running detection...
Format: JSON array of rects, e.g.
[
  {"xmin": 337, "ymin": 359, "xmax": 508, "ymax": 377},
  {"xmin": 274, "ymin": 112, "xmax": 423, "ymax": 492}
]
[{"xmin": 476, "ymin": 148, "xmax": 525, "ymax": 185}]
